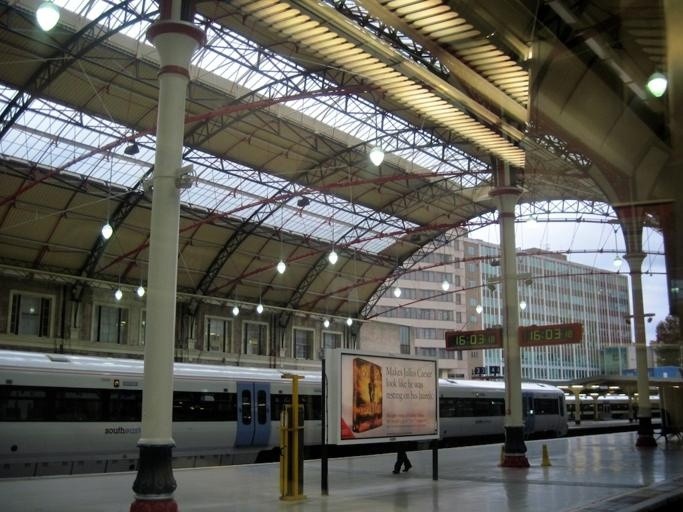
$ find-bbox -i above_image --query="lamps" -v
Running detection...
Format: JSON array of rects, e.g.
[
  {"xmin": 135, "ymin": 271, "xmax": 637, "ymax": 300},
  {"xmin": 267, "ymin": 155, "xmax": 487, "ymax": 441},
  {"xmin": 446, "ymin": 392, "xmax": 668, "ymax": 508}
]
[
  {"xmin": 612, "ymin": 224, "xmax": 624, "ymax": 266},
  {"xmin": 645, "ymin": 2, "xmax": 668, "ymax": 98}
]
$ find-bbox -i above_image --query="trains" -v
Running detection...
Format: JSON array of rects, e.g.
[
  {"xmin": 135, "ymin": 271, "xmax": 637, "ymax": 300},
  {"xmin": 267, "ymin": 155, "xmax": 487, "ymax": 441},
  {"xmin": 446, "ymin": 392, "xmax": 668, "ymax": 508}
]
[
  {"xmin": 564, "ymin": 392, "xmax": 664, "ymax": 422},
  {"xmin": 1, "ymin": 348, "xmax": 569, "ymax": 463}
]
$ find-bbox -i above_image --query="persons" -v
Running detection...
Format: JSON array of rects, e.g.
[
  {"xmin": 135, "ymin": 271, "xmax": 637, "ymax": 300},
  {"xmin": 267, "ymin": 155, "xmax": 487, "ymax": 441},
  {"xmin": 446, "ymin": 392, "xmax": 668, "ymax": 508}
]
[{"xmin": 392, "ymin": 441, "xmax": 411, "ymax": 474}]
